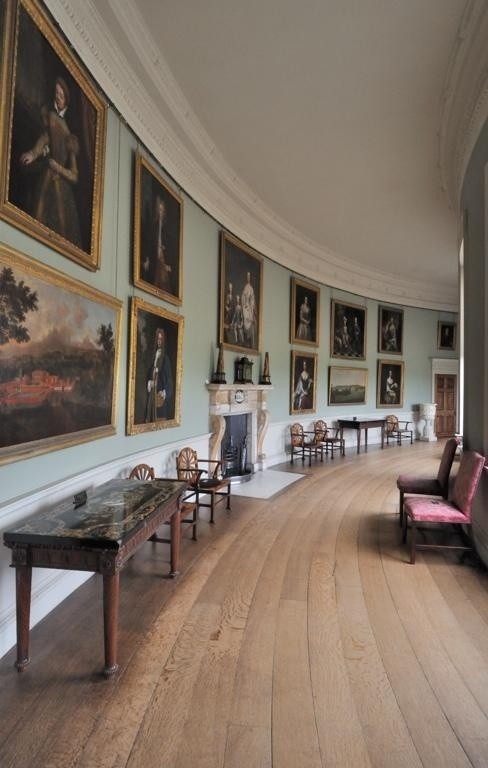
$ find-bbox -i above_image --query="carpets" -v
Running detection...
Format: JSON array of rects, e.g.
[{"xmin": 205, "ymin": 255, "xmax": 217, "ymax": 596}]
[{"xmin": 213, "ymin": 467, "xmax": 309, "ymax": 501}]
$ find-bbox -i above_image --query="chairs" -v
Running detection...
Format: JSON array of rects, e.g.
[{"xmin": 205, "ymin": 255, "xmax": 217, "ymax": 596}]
[
  {"xmin": 398, "ymin": 435, "xmax": 461, "ymax": 530},
  {"xmin": 129, "ymin": 464, "xmax": 197, "ymax": 579},
  {"xmin": 400, "ymin": 449, "xmax": 485, "ymax": 569},
  {"xmin": 176, "ymin": 448, "xmax": 233, "ymax": 526},
  {"xmin": 289, "ymin": 415, "xmax": 416, "ymax": 467}
]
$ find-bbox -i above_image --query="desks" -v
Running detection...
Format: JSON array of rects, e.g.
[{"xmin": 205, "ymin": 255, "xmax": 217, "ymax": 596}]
[{"xmin": 1, "ymin": 477, "xmax": 187, "ymax": 682}]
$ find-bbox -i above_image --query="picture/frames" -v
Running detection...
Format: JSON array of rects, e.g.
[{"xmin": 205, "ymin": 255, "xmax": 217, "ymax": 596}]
[
  {"xmin": 436, "ymin": 319, "xmax": 457, "ymax": 351},
  {"xmin": 3, "ymin": 2, "xmax": 110, "ymax": 272},
  {"xmin": 128, "ymin": 295, "xmax": 191, "ymax": 435},
  {"xmin": 2, "ymin": 244, "xmax": 122, "ymax": 465},
  {"xmin": 286, "ymin": 276, "xmax": 410, "ymax": 416},
  {"xmin": 129, "ymin": 147, "xmax": 186, "ymax": 313},
  {"xmin": 217, "ymin": 228, "xmax": 265, "ymax": 357}
]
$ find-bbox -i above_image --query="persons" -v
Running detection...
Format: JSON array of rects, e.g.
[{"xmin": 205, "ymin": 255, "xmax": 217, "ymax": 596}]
[
  {"xmin": 142, "ymin": 326, "xmax": 174, "ymax": 424},
  {"xmin": 334, "ymin": 314, "xmax": 353, "ymax": 349},
  {"xmin": 384, "ymin": 370, "xmax": 399, "ymax": 403},
  {"xmin": 384, "ymin": 315, "xmax": 397, "ymax": 351},
  {"xmin": 239, "ymin": 270, "xmax": 256, "ymax": 338},
  {"xmin": 18, "ymin": 75, "xmax": 85, "ymax": 251},
  {"xmin": 294, "ymin": 360, "xmax": 312, "ymax": 409},
  {"xmin": 350, "ymin": 316, "xmax": 362, "ymax": 353},
  {"xmin": 231, "ymin": 294, "xmax": 245, "ymax": 345},
  {"xmin": 296, "ymin": 296, "xmax": 313, "ymax": 342},
  {"xmin": 139, "ymin": 193, "xmax": 175, "ymax": 292},
  {"xmin": 225, "ymin": 281, "xmax": 235, "ymax": 330}
]
